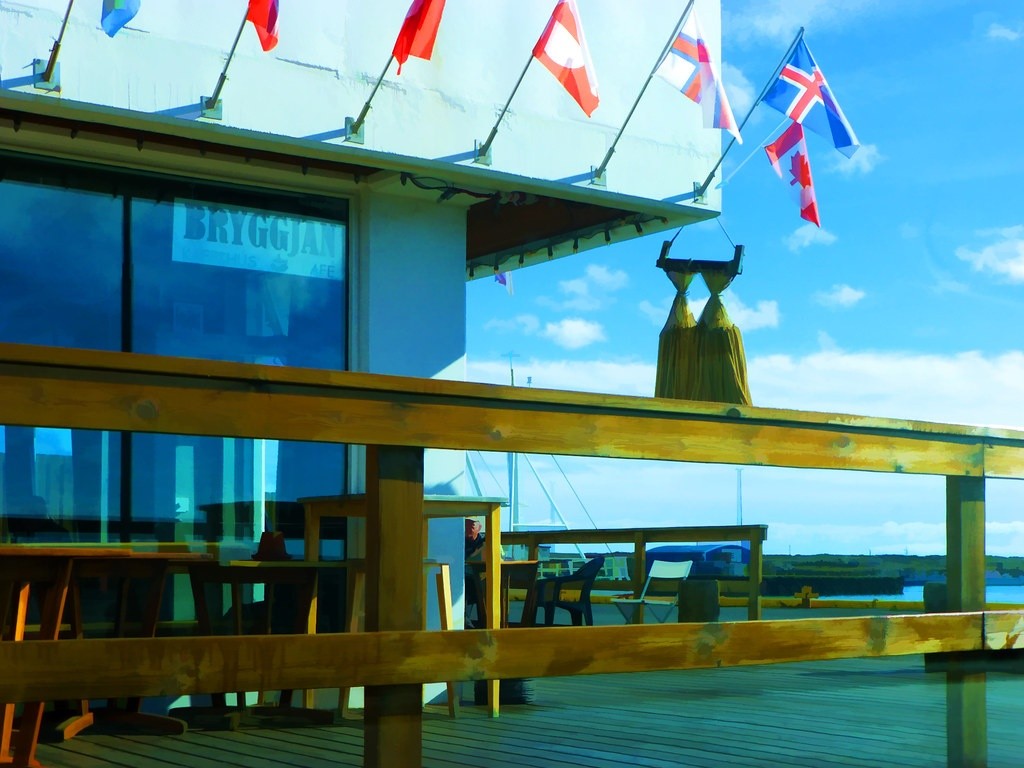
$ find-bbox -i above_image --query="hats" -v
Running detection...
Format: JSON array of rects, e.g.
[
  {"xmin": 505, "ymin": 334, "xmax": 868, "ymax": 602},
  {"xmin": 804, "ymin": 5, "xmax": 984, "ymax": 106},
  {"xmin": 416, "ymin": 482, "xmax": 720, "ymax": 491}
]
[{"xmin": 466, "ymin": 516, "xmax": 479, "ymax": 522}]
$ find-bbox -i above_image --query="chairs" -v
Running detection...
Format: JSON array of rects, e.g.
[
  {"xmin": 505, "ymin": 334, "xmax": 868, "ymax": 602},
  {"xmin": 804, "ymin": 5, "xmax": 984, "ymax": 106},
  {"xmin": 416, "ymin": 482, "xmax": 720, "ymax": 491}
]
[
  {"xmin": 531, "ymin": 556, "xmax": 606, "ymax": 626},
  {"xmin": 610, "ymin": 560, "xmax": 694, "ymax": 625}
]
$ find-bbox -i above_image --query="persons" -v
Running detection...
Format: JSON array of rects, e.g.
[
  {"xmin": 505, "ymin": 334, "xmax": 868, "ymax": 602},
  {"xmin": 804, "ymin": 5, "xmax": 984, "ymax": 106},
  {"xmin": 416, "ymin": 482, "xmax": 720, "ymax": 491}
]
[{"xmin": 463, "ymin": 514, "xmax": 507, "ymax": 629}]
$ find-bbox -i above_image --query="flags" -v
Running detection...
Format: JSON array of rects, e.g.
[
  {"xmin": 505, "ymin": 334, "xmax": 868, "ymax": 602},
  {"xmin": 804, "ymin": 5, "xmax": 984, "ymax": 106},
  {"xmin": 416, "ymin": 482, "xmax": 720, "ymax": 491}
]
[
  {"xmin": 763, "ymin": 120, "xmax": 821, "ymax": 228},
  {"xmin": 655, "ymin": 0, "xmax": 744, "ymax": 145},
  {"xmin": 532, "ymin": 0, "xmax": 599, "ymax": 118},
  {"xmin": 245, "ymin": 0, "xmax": 280, "ymax": 52},
  {"xmin": 391, "ymin": 0, "xmax": 447, "ymax": 75},
  {"xmin": 101, "ymin": 0, "xmax": 142, "ymax": 38},
  {"xmin": 762, "ymin": 32, "xmax": 860, "ymax": 159}
]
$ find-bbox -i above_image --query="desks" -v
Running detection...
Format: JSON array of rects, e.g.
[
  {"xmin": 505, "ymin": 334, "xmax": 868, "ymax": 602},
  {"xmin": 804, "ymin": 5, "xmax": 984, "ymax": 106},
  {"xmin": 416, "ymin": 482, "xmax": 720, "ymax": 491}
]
[
  {"xmin": 0, "ymin": 546, "xmax": 135, "ymax": 768},
  {"xmin": 169, "ymin": 558, "xmax": 461, "ymax": 730},
  {"xmin": 297, "ymin": 493, "xmax": 510, "ymax": 719},
  {"xmin": 51, "ymin": 551, "xmax": 213, "ymax": 741},
  {"xmin": 462, "ymin": 560, "xmax": 544, "ymax": 628}
]
